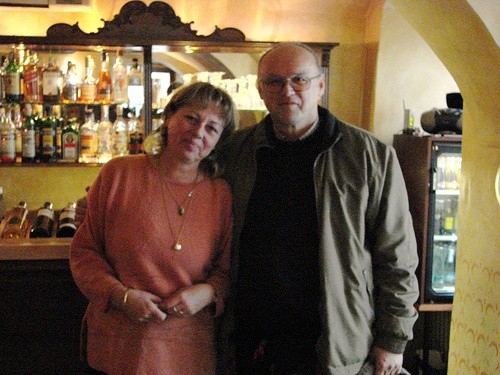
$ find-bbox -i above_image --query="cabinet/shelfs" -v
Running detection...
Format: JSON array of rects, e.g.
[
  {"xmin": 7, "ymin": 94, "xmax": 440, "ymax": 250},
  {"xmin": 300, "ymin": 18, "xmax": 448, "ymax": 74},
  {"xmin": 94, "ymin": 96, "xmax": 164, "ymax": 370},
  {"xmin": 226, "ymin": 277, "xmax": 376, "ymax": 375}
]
[{"xmin": 0, "ymin": 35, "xmax": 341, "ymax": 168}]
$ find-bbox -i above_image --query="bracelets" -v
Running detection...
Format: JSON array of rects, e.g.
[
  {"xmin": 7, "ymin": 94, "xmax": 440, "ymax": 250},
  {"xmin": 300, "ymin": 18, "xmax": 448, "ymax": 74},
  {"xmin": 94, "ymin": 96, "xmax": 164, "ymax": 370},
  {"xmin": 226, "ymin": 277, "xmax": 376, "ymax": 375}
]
[{"xmin": 118, "ymin": 287, "xmax": 129, "ymax": 313}]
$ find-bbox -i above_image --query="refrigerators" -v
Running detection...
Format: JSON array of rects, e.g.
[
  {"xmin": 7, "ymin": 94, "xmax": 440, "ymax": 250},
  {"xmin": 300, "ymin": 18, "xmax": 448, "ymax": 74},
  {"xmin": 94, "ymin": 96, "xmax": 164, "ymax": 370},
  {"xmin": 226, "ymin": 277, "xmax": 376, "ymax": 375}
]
[{"xmin": 393, "ymin": 134, "xmax": 463, "ymax": 307}]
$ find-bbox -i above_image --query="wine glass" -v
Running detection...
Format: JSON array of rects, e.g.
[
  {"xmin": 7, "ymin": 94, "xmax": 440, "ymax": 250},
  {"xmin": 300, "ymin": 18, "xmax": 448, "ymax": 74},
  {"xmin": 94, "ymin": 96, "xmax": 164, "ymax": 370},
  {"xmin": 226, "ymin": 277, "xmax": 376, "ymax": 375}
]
[{"xmin": 152, "ymin": 71, "xmax": 267, "ymax": 109}]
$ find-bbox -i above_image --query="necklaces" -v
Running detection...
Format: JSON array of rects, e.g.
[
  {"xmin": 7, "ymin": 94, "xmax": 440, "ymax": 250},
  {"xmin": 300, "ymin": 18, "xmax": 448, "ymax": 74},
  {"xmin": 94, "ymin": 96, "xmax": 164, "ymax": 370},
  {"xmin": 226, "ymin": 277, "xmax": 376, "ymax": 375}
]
[{"xmin": 158, "ymin": 156, "xmax": 200, "ymax": 250}]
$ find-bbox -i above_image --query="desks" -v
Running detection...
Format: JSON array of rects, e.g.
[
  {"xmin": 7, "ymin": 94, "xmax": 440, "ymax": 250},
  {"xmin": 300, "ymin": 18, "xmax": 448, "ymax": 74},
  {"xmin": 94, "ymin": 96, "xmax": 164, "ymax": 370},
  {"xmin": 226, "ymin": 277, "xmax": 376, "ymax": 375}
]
[{"xmin": 0, "ymin": 219, "xmax": 90, "ymax": 375}]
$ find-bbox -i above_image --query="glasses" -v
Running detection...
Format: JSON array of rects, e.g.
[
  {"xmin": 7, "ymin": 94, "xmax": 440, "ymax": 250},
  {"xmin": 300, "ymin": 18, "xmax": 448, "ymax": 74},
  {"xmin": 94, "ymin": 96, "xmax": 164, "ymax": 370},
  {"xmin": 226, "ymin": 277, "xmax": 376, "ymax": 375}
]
[{"xmin": 260, "ymin": 72, "xmax": 322, "ymax": 94}]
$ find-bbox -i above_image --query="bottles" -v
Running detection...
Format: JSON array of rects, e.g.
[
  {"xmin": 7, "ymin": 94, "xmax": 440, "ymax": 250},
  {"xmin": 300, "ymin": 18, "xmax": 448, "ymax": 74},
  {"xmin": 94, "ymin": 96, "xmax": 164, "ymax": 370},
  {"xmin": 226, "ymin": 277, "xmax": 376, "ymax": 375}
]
[
  {"xmin": 29, "ymin": 201, "xmax": 54, "ymax": 239},
  {"xmin": 0, "ymin": 43, "xmax": 146, "ymax": 162},
  {"xmin": 1, "ymin": 201, "xmax": 29, "ymax": 238},
  {"xmin": 433, "ymin": 152, "xmax": 464, "ymax": 288},
  {"xmin": 56, "ymin": 202, "xmax": 77, "ymax": 238}
]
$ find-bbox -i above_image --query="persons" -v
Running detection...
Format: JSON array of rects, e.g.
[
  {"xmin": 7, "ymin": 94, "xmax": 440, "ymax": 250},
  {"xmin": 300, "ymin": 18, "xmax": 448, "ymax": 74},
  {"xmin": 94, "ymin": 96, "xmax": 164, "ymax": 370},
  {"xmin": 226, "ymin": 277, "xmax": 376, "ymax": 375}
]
[
  {"xmin": 69, "ymin": 82, "xmax": 239, "ymax": 375},
  {"xmin": 73, "ymin": 41, "xmax": 419, "ymax": 375}
]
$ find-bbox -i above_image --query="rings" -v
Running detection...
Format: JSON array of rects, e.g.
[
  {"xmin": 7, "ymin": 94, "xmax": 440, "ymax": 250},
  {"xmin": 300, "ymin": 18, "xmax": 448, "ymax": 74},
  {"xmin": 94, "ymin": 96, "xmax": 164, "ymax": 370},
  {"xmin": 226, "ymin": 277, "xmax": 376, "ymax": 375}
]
[
  {"xmin": 179, "ymin": 310, "xmax": 183, "ymax": 314},
  {"xmin": 173, "ymin": 307, "xmax": 177, "ymax": 312}
]
